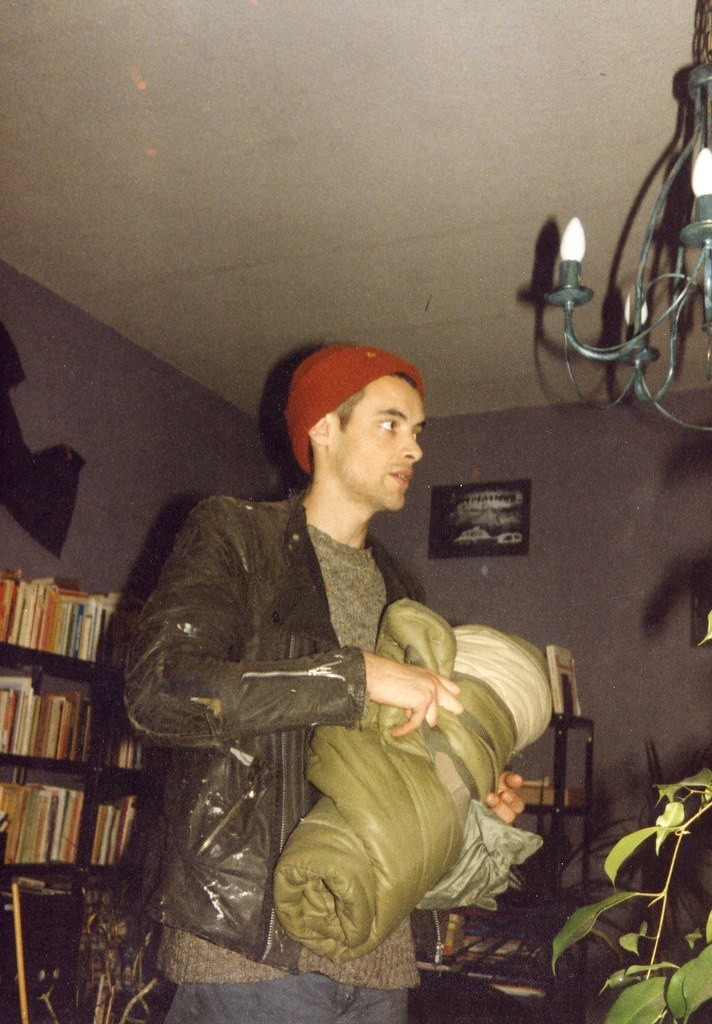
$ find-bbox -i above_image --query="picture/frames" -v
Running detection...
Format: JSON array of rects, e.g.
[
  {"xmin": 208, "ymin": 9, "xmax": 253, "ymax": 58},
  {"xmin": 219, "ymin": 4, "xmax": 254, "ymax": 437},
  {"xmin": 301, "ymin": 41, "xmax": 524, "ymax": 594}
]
[{"xmin": 427, "ymin": 479, "xmax": 533, "ymax": 558}]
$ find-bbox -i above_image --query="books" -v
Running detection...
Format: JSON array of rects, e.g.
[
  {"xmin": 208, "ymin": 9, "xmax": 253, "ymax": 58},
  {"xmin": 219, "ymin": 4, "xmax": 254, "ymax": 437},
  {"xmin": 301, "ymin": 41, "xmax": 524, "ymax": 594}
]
[{"xmin": 1, "ymin": 573, "xmax": 569, "ymax": 997}]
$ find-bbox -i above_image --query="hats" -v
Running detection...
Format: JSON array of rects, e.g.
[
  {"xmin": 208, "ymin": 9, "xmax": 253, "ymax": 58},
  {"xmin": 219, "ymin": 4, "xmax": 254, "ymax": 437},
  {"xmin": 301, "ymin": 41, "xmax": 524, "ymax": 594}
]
[{"xmin": 287, "ymin": 343, "xmax": 425, "ymax": 473}]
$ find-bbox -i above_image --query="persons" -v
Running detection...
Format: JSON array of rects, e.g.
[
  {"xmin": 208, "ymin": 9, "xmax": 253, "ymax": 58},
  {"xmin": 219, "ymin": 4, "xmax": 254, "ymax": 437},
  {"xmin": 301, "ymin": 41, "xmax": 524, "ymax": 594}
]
[{"xmin": 121, "ymin": 344, "xmax": 524, "ymax": 1024}]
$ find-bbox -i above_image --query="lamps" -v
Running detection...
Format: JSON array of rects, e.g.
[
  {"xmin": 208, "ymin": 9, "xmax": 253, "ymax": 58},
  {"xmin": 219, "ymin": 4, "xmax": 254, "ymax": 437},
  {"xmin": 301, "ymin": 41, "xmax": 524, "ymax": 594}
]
[{"xmin": 546, "ymin": 0, "xmax": 712, "ymax": 436}]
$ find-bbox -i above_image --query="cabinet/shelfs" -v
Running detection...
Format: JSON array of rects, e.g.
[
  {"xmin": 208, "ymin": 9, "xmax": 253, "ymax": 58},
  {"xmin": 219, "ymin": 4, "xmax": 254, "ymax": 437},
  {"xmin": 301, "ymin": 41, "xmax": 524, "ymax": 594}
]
[
  {"xmin": 0, "ymin": 639, "xmax": 172, "ymax": 1024},
  {"xmin": 414, "ymin": 715, "xmax": 596, "ymax": 1024}
]
